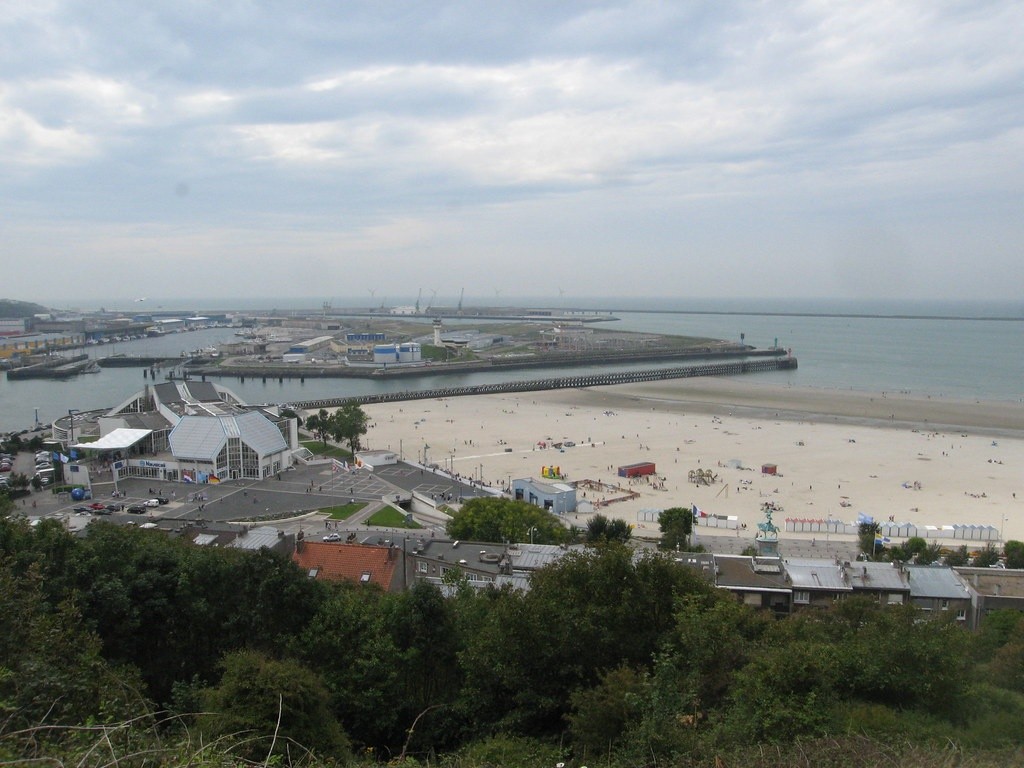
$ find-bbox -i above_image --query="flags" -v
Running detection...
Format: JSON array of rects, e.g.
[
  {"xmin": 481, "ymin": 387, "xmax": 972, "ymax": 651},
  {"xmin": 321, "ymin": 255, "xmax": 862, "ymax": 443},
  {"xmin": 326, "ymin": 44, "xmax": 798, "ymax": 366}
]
[
  {"xmin": 875, "ymin": 533, "xmax": 891, "ymax": 545},
  {"xmin": 342, "ymin": 461, "xmax": 350, "ymax": 472},
  {"xmin": 60, "ymin": 452, "xmax": 69, "ymax": 463},
  {"xmin": 71, "ymin": 450, "xmax": 79, "ymax": 458},
  {"xmin": 693, "ymin": 505, "xmax": 708, "ymax": 518},
  {"xmin": 354, "ymin": 457, "xmax": 362, "ymax": 467},
  {"xmin": 53, "ymin": 452, "xmax": 59, "ymax": 461},
  {"xmin": 209, "ymin": 474, "xmax": 220, "ymax": 484},
  {"xmin": 183, "ymin": 472, "xmax": 193, "ymax": 484},
  {"xmin": 331, "ymin": 463, "xmax": 339, "ymax": 472}
]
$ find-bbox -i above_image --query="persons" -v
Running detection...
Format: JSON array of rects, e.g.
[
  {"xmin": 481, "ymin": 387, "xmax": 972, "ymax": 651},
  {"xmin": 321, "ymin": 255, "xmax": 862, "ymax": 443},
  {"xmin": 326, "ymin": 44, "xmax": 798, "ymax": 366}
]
[{"xmin": 11, "ymin": 392, "xmax": 1023, "ymax": 567}]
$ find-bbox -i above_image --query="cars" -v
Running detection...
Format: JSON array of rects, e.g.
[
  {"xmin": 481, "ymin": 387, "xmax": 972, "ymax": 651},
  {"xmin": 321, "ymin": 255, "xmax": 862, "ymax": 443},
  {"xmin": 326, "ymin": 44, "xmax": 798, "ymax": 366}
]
[
  {"xmin": 322, "ymin": 533, "xmax": 342, "ymax": 542},
  {"xmin": 72, "ymin": 495, "xmax": 169, "ymax": 518},
  {"xmin": 0, "ymin": 448, "xmax": 55, "ymax": 488}
]
